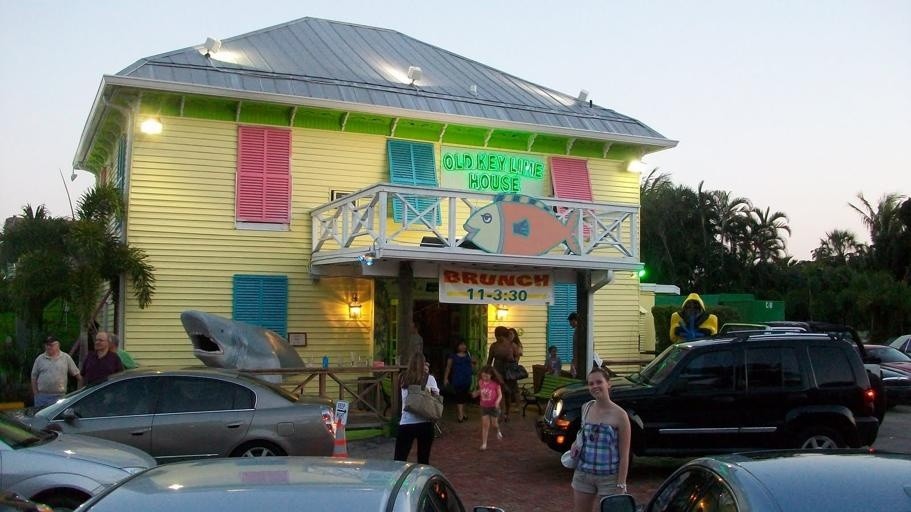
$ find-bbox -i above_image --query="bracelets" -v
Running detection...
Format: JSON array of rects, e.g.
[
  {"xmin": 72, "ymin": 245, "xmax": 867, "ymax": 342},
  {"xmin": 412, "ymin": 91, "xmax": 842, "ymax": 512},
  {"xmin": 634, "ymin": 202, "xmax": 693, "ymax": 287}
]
[{"xmin": 616, "ymin": 483, "xmax": 626, "ymax": 489}]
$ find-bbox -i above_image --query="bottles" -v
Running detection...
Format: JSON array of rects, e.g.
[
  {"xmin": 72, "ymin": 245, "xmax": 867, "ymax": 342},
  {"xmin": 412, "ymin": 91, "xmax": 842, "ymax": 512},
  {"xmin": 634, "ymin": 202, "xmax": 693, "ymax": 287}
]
[{"xmin": 323, "ymin": 354, "xmax": 329, "ymax": 369}]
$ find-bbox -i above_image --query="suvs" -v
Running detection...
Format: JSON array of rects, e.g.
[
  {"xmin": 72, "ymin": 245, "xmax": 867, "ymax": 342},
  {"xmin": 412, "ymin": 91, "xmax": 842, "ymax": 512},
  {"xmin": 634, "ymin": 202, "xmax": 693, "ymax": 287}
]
[{"xmin": 533, "ymin": 325, "xmax": 889, "ymax": 457}]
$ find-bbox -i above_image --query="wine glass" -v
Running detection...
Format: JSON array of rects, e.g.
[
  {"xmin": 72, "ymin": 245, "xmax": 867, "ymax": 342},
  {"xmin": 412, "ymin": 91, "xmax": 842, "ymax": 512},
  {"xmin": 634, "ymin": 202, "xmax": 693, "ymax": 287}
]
[{"xmin": 348, "ymin": 351, "xmax": 372, "ymax": 367}]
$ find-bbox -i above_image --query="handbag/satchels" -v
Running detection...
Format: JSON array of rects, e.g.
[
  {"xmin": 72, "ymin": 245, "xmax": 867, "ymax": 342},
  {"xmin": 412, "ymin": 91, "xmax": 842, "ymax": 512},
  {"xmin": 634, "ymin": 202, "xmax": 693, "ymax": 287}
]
[
  {"xmin": 560, "ymin": 399, "xmax": 597, "ymax": 470},
  {"xmin": 403, "ymin": 383, "xmax": 446, "ymax": 421},
  {"xmin": 505, "ymin": 363, "xmax": 530, "ymax": 382}
]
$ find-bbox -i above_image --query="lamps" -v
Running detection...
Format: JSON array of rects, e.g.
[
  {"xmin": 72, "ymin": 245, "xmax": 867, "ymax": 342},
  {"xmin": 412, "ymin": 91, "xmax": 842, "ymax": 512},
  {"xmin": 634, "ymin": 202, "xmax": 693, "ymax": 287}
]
[
  {"xmin": 362, "ymin": 236, "xmax": 379, "ymax": 259},
  {"xmin": 578, "ymin": 89, "xmax": 588, "ymax": 106},
  {"xmin": 408, "ymin": 66, "xmax": 421, "ymax": 86},
  {"xmin": 205, "ymin": 37, "xmax": 221, "ymax": 57},
  {"xmin": 142, "ymin": 117, "xmax": 161, "ymax": 133},
  {"xmin": 71, "ymin": 169, "xmax": 77, "ymax": 181},
  {"xmin": 496, "ymin": 304, "xmax": 508, "ymax": 319},
  {"xmin": 624, "ymin": 159, "xmax": 641, "ymax": 171},
  {"xmin": 349, "ymin": 293, "xmax": 362, "ymax": 318}
]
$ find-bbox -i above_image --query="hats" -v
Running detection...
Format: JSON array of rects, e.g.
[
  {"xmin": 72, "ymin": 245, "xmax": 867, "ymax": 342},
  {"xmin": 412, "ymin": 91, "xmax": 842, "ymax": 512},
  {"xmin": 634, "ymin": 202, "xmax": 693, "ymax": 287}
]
[{"xmin": 41, "ymin": 333, "xmax": 63, "ymax": 344}]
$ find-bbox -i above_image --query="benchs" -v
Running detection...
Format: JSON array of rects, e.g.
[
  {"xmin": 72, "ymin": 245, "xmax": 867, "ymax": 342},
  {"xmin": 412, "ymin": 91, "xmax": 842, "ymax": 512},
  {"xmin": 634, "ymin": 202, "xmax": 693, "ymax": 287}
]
[{"xmin": 522, "ymin": 373, "xmax": 585, "ymax": 416}]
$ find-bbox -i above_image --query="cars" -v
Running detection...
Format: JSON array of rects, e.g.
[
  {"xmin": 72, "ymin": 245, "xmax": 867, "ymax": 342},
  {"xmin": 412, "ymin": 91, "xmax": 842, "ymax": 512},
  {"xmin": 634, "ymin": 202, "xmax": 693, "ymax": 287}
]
[
  {"xmin": 598, "ymin": 447, "xmax": 911, "ymax": 511},
  {"xmin": 851, "ymin": 330, "xmax": 911, "ymax": 406}
]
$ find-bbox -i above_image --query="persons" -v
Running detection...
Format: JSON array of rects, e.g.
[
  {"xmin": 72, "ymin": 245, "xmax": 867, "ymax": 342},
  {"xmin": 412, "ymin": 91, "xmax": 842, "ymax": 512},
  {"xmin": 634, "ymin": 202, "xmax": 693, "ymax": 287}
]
[
  {"xmin": 31, "ymin": 331, "xmax": 138, "ymax": 415},
  {"xmin": 394, "ymin": 310, "xmax": 595, "ymax": 464},
  {"xmin": 569, "ymin": 367, "xmax": 631, "ymax": 512}
]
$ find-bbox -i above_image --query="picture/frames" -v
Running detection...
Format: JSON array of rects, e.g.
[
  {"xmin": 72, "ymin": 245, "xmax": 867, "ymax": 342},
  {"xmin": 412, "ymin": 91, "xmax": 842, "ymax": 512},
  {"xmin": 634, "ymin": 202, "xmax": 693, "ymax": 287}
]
[{"xmin": 289, "ymin": 332, "xmax": 307, "ymax": 347}]
